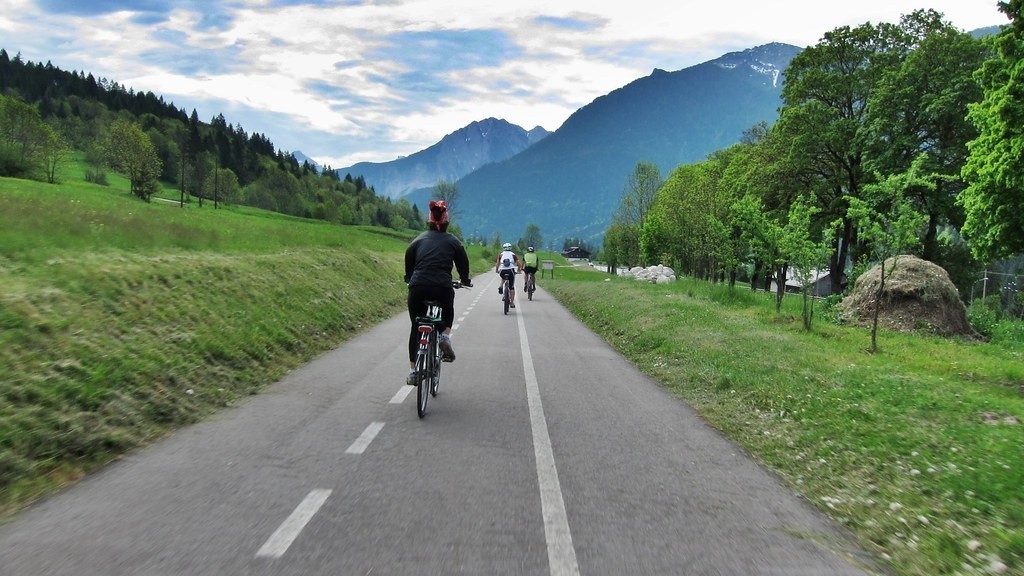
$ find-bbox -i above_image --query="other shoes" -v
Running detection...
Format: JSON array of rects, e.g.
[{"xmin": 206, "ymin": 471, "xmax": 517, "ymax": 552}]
[
  {"xmin": 533, "ymin": 288, "xmax": 536, "ymax": 292},
  {"xmin": 524, "ymin": 285, "xmax": 528, "ymax": 292},
  {"xmin": 498, "ymin": 287, "xmax": 503, "ymax": 294},
  {"xmin": 510, "ymin": 304, "xmax": 515, "ymax": 308},
  {"xmin": 407, "ymin": 372, "xmax": 417, "ymax": 385},
  {"xmin": 439, "ymin": 334, "xmax": 455, "ymax": 360}
]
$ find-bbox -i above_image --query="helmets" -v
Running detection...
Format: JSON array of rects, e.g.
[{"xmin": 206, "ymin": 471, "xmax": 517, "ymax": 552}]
[
  {"xmin": 527, "ymin": 247, "xmax": 534, "ymax": 252},
  {"xmin": 503, "ymin": 243, "xmax": 512, "ymax": 251}
]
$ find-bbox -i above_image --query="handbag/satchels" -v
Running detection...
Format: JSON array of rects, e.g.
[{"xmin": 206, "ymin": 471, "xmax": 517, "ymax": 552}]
[{"xmin": 501, "ymin": 259, "xmax": 513, "ymax": 266}]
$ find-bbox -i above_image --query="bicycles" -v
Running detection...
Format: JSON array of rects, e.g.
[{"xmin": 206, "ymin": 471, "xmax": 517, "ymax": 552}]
[
  {"xmin": 521, "ymin": 268, "xmax": 539, "ymax": 301},
  {"xmin": 405, "ymin": 280, "xmax": 473, "ymax": 418},
  {"xmin": 496, "ymin": 271, "xmax": 522, "ymax": 315}
]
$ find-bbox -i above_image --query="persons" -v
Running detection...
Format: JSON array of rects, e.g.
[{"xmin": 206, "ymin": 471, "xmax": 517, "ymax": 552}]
[
  {"xmin": 496, "ymin": 242, "xmax": 522, "ymax": 307},
  {"xmin": 403, "ymin": 201, "xmax": 472, "ymax": 385},
  {"xmin": 522, "ymin": 245, "xmax": 539, "ymax": 292}
]
